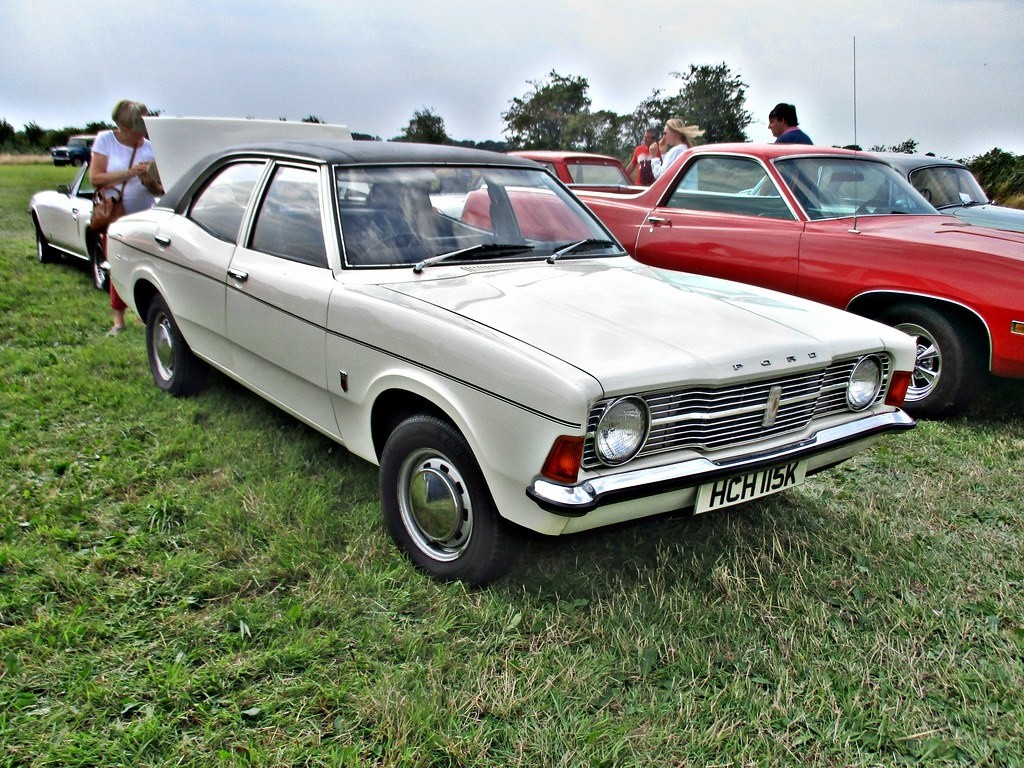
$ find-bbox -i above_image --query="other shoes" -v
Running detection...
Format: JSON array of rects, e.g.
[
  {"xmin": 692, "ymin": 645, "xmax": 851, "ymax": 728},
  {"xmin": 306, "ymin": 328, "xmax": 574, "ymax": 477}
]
[{"xmin": 105, "ymin": 327, "xmax": 126, "ymax": 337}]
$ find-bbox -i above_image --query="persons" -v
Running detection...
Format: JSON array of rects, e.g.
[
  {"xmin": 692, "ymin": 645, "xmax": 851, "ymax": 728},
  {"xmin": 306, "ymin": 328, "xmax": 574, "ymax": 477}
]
[
  {"xmin": 89, "ymin": 99, "xmax": 166, "ymax": 338},
  {"xmin": 758, "ymin": 103, "xmax": 822, "ymax": 210},
  {"xmin": 625, "ymin": 128, "xmax": 662, "ymax": 187},
  {"xmin": 649, "ymin": 118, "xmax": 706, "ymax": 191}
]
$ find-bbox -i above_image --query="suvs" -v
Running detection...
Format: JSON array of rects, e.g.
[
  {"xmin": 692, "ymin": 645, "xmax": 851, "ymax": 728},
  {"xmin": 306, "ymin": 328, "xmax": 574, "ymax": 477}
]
[{"xmin": 50, "ymin": 136, "xmax": 97, "ymax": 167}]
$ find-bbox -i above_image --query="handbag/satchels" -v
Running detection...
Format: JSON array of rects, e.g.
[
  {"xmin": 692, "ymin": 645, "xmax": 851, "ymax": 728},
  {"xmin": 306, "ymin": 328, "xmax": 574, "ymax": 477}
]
[{"xmin": 91, "ymin": 186, "xmax": 125, "ymax": 234}]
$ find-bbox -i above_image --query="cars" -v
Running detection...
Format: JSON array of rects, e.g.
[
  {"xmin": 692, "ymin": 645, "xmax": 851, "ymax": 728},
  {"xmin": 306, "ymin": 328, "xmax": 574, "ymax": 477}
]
[
  {"xmin": 102, "ymin": 115, "xmax": 919, "ymax": 588},
  {"xmin": 28, "ymin": 161, "xmax": 111, "ymax": 291},
  {"xmin": 427, "ymin": 151, "xmax": 634, "ymax": 224},
  {"xmin": 462, "ymin": 142, "xmax": 1024, "ymax": 419},
  {"xmin": 735, "ymin": 150, "xmax": 1024, "ymax": 234}
]
